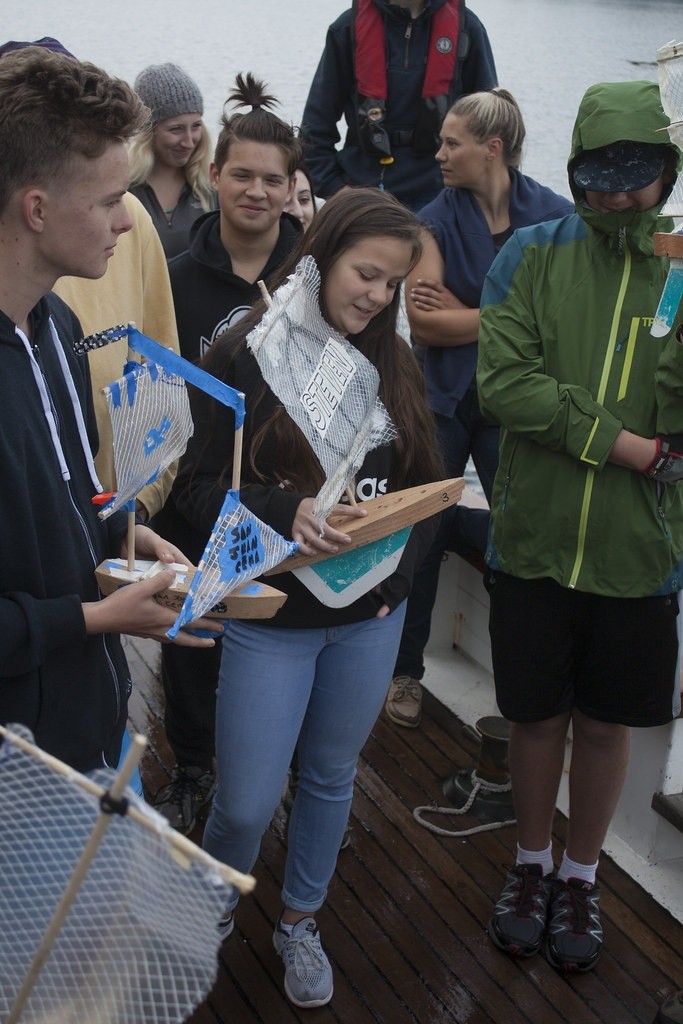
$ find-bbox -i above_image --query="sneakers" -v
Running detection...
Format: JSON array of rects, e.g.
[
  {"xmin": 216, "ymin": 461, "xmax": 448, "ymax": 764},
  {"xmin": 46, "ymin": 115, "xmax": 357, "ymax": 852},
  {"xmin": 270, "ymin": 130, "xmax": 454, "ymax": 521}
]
[
  {"xmin": 487, "ymin": 858, "xmax": 558, "ymax": 959},
  {"xmin": 153, "ymin": 764, "xmax": 218, "ymax": 838},
  {"xmin": 288, "ymin": 771, "xmax": 352, "ymax": 850},
  {"xmin": 544, "ymin": 876, "xmax": 605, "ymax": 973},
  {"xmin": 215, "ymin": 909, "xmax": 235, "ymax": 943},
  {"xmin": 271, "ymin": 907, "xmax": 334, "ymax": 1009}
]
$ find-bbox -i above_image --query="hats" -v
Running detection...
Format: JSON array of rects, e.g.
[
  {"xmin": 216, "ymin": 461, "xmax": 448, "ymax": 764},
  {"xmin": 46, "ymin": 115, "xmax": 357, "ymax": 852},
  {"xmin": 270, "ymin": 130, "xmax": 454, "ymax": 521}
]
[
  {"xmin": 0, "ymin": 36, "xmax": 79, "ymax": 62},
  {"xmin": 134, "ymin": 63, "xmax": 203, "ymax": 124},
  {"xmin": 573, "ymin": 139, "xmax": 671, "ymax": 192}
]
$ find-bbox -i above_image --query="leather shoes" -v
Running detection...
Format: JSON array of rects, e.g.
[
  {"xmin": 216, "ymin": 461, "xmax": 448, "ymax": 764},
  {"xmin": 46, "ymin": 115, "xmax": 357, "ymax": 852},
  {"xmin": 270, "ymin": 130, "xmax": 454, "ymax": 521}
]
[{"xmin": 385, "ymin": 676, "xmax": 423, "ymax": 728}]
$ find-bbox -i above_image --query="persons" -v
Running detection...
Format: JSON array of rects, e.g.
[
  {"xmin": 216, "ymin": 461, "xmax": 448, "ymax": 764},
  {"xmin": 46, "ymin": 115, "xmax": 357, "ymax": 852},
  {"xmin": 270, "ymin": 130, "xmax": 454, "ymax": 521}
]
[
  {"xmin": 0, "ymin": 34, "xmax": 203, "ymax": 544},
  {"xmin": 0, "ymin": 45, "xmax": 153, "ymax": 809},
  {"xmin": 127, "ymin": 58, "xmax": 224, "ymax": 271},
  {"xmin": 165, "ymin": 185, "xmax": 458, "ymax": 1008},
  {"xmin": 386, "ymin": 86, "xmax": 583, "ymax": 729},
  {"xmin": 472, "ymin": 79, "xmax": 683, "ymax": 979},
  {"xmin": 289, "ymin": 0, "xmax": 502, "ymax": 219},
  {"xmin": 147, "ymin": 71, "xmax": 312, "ymax": 839}
]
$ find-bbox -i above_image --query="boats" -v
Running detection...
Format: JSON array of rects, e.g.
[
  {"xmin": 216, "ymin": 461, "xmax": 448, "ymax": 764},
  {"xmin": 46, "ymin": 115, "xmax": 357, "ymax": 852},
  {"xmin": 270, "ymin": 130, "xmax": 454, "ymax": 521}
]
[
  {"xmin": 71, "ymin": 322, "xmax": 288, "ymax": 622},
  {"xmin": 166, "ymin": 461, "xmax": 465, "ymax": 642}
]
